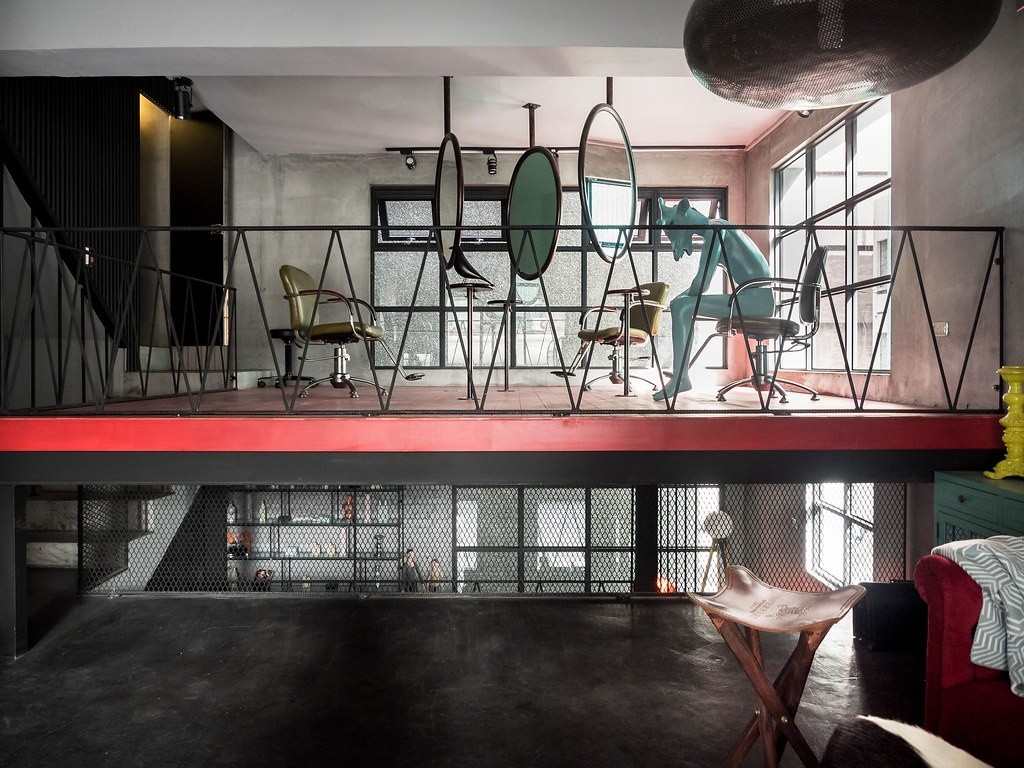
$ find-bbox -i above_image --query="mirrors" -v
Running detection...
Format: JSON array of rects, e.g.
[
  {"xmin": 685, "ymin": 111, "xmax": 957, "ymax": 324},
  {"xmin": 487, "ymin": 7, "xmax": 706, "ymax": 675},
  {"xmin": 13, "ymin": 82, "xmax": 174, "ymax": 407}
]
[
  {"xmin": 432, "ymin": 75, "xmax": 464, "ymax": 271},
  {"xmin": 504, "ymin": 102, "xmax": 562, "ymax": 282},
  {"xmin": 577, "ymin": 77, "xmax": 639, "ymax": 265}
]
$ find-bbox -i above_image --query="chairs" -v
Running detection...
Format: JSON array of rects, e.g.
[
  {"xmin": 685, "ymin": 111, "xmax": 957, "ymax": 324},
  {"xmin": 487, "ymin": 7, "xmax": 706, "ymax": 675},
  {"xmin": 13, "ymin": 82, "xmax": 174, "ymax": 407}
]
[
  {"xmin": 279, "ymin": 264, "xmax": 425, "ymax": 400},
  {"xmin": 913, "ymin": 535, "xmax": 1024, "ymax": 768},
  {"xmin": 549, "ymin": 281, "xmax": 671, "ymax": 391},
  {"xmin": 662, "ymin": 246, "xmax": 831, "ymax": 402}
]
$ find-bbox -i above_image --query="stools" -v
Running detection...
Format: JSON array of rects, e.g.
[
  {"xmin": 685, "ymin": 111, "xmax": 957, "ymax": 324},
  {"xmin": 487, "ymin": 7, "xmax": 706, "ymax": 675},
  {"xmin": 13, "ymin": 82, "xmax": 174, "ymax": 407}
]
[
  {"xmin": 604, "ymin": 289, "xmax": 651, "ymax": 397},
  {"xmin": 258, "ymin": 329, "xmax": 319, "ymax": 389},
  {"xmin": 684, "ymin": 563, "xmax": 868, "ymax": 768},
  {"xmin": 446, "ymin": 283, "xmax": 495, "ymax": 401},
  {"xmin": 487, "ymin": 300, "xmax": 524, "ymax": 392}
]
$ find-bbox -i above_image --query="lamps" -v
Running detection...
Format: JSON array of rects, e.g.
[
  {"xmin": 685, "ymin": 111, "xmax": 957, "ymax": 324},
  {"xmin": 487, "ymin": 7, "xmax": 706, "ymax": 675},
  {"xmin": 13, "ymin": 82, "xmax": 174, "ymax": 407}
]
[
  {"xmin": 705, "ymin": 511, "xmax": 732, "ymax": 544},
  {"xmin": 400, "ymin": 149, "xmax": 417, "ymax": 170},
  {"xmin": 172, "ymin": 75, "xmax": 194, "ymax": 120},
  {"xmin": 483, "ymin": 150, "xmax": 497, "ymax": 175},
  {"xmin": 798, "ymin": 110, "xmax": 815, "ymax": 118},
  {"xmin": 84, "ymin": 246, "xmax": 98, "ymax": 268}
]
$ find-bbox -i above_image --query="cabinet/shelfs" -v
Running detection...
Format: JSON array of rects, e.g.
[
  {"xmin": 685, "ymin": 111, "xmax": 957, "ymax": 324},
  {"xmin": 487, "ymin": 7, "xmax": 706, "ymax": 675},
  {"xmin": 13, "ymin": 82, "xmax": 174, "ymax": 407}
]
[{"xmin": 224, "ymin": 486, "xmax": 406, "ymax": 593}]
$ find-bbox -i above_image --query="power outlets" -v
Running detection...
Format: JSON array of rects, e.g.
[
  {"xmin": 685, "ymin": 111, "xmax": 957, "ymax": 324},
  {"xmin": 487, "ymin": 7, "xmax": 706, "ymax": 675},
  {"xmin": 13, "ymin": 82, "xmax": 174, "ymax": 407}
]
[{"xmin": 931, "ymin": 322, "xmax": 949, "ymax": 337}]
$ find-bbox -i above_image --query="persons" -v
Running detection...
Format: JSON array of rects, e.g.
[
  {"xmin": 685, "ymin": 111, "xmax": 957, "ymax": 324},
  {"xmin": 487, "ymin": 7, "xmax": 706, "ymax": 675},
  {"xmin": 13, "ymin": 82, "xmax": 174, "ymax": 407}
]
[
  {"xmin": 653, "ymin": 198, "xmax": 776, "ymax": 401},
  {"xmin": 251, "ymin": 569, "xmax": 271, "ymax": 594},
  {"xmin": 399, "ymin": 549, "xmax": 426, "ymax": 594},
  {"xmin": 425, "ymin": 559, "xmax": 444, "ymax": 593}
]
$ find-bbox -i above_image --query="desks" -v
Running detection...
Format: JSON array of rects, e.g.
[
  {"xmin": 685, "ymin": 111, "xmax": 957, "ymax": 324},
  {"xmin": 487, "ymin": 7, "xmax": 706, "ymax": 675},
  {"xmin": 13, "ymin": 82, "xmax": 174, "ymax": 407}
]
[{"xmin": 933, "ymin": 469, "xmax": 1024, "ymax": 550}]
